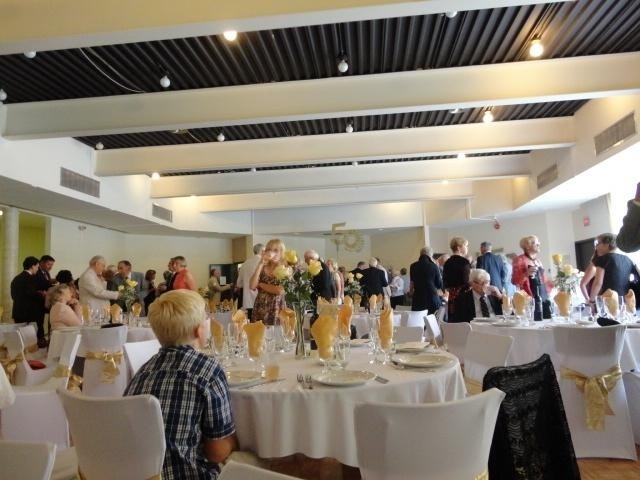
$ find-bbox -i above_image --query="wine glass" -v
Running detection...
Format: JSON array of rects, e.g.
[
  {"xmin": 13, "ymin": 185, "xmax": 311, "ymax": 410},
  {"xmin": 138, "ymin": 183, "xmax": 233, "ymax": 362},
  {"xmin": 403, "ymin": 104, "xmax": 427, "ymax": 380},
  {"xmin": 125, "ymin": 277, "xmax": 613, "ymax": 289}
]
[
  {"xmin": 82, "ymin": 308, "xmax": 140, "ymax": 327},
  {"xmin": 209, "ymin": 321, "xmax": 295, "ymax": 382},
  {"xmin": 317, "ymin": 315, "xmax": 350, "ymax": 375},
  {"xmin": 501, "ymin": 297, "xmax": 535, "ymax": 325},
  {"xmin": 369, "ymin": 304, "xmax": 382, "ymax": 315},
  {"xmin": 594, "ymin": 295, "xmax": 636, "ymax": 324},
  {"xmin": 344, "ymin": 302, "xmax": 360, "ymax": 313},
  {"xmin": 367, "ymin": 316, "xmax": 395, "ymax": 367}
]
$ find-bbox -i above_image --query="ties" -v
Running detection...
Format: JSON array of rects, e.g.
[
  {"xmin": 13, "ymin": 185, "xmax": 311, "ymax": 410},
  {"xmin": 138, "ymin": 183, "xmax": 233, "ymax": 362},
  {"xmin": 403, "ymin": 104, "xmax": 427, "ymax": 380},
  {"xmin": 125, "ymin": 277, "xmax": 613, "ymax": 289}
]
[{"xmin": 480, "ymin": 296, "xmax": 490, "ymax": 317}]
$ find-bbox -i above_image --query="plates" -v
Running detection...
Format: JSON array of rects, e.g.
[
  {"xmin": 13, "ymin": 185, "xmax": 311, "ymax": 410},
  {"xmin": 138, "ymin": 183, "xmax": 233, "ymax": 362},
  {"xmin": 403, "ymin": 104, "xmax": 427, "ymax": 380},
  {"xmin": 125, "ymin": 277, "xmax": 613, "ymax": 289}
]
[
  {"xmin": 473, "ymin": 316, "xmax": 498, "ymax": 322},
  {"xmin": 394, "ymin": 342, "xmax": 434, "ymax": 352},
  {"xmin": 493, "ymin": 321, "xmax": 518, "ymax": 326},
  {"xmin": 391, "ymin": 352, "xmax": 455, "ymax": 367},
  {"xmin": 225, "ymin": 369, "xmax": 262, "ymax": 387},
  {"xmin": 311, "ymin": 368, "xmax": 378, "ymax": 386}
]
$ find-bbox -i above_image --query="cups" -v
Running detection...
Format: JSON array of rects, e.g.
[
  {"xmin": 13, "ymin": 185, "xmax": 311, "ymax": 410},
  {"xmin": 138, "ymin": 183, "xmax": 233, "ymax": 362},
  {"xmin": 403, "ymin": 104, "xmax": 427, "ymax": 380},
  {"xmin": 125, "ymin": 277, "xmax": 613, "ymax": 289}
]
[
  {"xmin": 482, "ymin": 284, "xmax": 491, "ymax": 295},
  {"xmin": 568, "ymin": 306, "xmax": 592, "ymax": 325}
]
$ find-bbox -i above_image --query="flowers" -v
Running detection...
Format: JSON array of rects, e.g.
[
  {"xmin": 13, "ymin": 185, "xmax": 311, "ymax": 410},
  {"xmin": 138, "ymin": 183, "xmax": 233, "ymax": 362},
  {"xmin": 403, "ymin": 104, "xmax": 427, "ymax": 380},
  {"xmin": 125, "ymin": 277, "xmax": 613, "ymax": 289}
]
[
  {"xmin": 348, "ymin": 272, "xmax": 363, "ymax": 305},
  {"xmin": 118, "ymin": 279, "xmax": 138, "ymax": 312},
  {"xmin": 274, "ymin": 250, "xmax": 323, "ymax": 354},
  {"xmin": 552, "ymin": 255, "xmax": 577, "ymax": 294}
]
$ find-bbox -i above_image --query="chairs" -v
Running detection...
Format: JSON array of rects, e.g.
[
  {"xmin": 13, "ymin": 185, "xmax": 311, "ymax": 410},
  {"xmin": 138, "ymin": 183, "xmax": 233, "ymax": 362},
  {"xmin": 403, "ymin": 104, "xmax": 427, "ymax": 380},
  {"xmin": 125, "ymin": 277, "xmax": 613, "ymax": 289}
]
[{"xmin": 0, "ymin": 305, "xmax": 640, "ymax": 480}]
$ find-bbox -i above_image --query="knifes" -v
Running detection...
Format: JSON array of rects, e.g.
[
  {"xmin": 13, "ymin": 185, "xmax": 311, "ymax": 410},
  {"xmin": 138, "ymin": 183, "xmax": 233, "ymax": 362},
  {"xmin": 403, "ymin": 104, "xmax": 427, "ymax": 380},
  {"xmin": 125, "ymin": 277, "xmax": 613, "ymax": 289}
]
[{"xmin": 239, "ymin": 379, "xmax": 285, "ymax": 389}]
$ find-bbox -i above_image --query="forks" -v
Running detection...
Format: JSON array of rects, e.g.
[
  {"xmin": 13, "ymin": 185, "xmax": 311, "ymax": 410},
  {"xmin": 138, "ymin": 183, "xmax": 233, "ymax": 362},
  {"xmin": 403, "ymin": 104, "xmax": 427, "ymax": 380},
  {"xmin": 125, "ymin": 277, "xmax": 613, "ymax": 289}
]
[{"xmin": 296, "ymin": 373, "xmax": 312, "ymax": 390}]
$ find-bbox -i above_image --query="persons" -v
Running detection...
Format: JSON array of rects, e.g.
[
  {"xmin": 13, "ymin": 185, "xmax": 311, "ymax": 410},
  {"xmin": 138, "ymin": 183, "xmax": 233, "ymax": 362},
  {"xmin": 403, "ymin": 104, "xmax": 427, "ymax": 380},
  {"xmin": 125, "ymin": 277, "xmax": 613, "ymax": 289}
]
[
  {"xmin": 11, "ymin": 254, "xmax": 194, "ymax": 347},
  {"xmin": 209, "ymin": 235, "xmax": 640, "ymax": 346},
  {"xmin": 614, "ymin": 182, "xmax": 640, "ymax": 253},
  {"xmin": 119, "ymin": 288, "xmax": 268, "ymax": 479}
]
